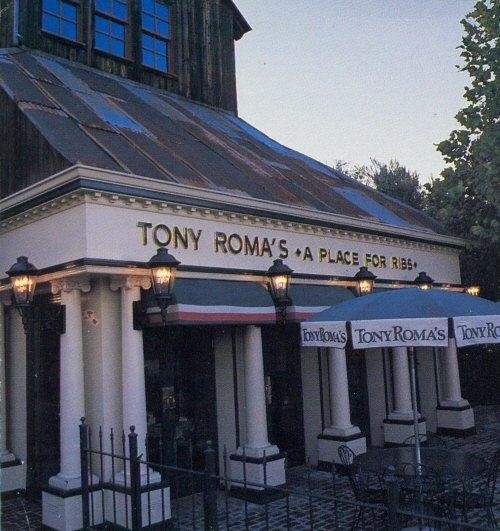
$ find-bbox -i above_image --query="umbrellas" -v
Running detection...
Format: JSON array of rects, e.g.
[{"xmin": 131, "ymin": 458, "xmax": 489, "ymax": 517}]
[{"xmin": 300, "ymin": 286, "xmax": 500, "ymax": 475}]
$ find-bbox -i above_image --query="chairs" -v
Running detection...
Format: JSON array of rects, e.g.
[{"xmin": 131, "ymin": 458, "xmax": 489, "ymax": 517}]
[{"xmin": 339, "ymin": 434, "xmax": 500, "ymax": 531}]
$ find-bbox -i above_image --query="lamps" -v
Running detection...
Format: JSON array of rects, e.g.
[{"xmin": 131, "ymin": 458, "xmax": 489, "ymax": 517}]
[
  {"xmin": 5, "ymin": 255, "xmax": 66, "ymax": 336},
  {"xmin": 132, "ymin": 247, "xmax": 182, "ymax": 331},
  {"xmin": 354, "ymin": 267, "xmax": 378, "ymax": 297},
  {"xmin": 255, "ymin": 258, "xmax": 294, "ymax": 334}
]
